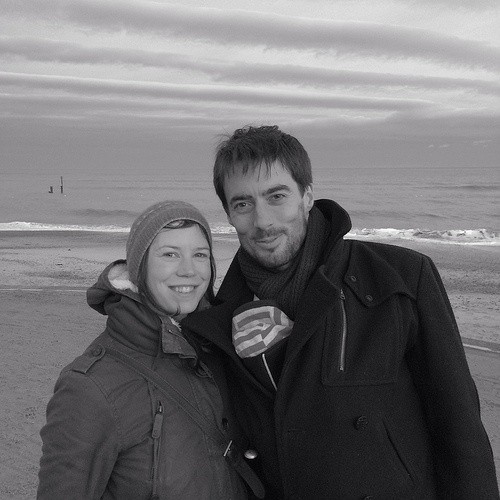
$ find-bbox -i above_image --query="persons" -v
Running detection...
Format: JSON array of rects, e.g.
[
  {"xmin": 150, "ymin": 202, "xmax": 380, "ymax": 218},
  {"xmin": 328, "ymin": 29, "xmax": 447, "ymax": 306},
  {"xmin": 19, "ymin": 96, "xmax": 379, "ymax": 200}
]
[
  {"xmin": 179, "ymin": 122, "xmax": 500, "ymax": 500},
  {"xmin": 39, "ymin": 199, "xmax": 267, "ymax": 500}
]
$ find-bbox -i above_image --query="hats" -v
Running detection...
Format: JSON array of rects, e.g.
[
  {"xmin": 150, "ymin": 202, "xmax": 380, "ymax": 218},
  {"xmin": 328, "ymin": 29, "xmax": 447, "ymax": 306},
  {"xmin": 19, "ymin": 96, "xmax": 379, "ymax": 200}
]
[{"xmin": 126, "ymin": 200, "xmax": 212, "ymax": 282}]
